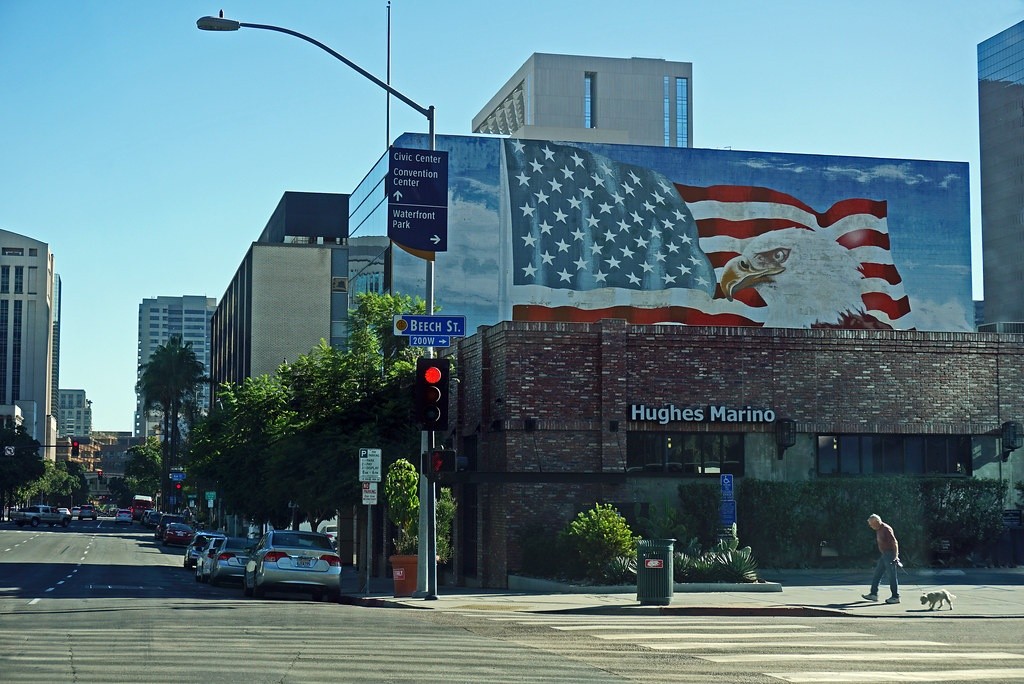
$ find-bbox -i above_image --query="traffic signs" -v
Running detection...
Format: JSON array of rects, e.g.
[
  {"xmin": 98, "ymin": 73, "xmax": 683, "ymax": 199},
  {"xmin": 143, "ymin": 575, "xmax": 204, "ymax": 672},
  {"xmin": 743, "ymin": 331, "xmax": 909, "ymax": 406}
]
[
  {"xmin": 169, "ymin": 465, "xmax": 186, "ymax": 489},
  {"xmin": 198, "ymin": 8, "xmax": 467, "ymax": 597}
]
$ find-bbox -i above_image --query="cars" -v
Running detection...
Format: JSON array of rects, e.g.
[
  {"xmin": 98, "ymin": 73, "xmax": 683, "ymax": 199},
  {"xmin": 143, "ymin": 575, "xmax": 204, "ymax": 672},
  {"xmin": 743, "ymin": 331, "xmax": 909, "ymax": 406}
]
[
  {"xmin": 140, "ymin": 510, "xmax": 162, "ymax": 528},
  {"xmin": 243, "ymin": 530, "xmax": 342, "ymax": 597},
  {"xmin": 163, "ymin": 524, "xmax": 194, "ymax": 546},
  {"xmin": 184, "ymin": 531, "xmax": 226, "ymax": 569},
  {"xmin": 57, "ymin": 508, "xmax": 70, "ymax": 515},
  {"xmin": 209, "ymin": 537, "xmax": 262, "ymax": 585},
  {"xmin": 71, "ymin": 507, "xmax": 81, "ymax": 515},
  {"xmin": 196, "ymin": 538, "xmax": 225, "ymax": 584},
  {"xmin": 320, "ymin": 525, "xmax": 338, "ymax": 542}
]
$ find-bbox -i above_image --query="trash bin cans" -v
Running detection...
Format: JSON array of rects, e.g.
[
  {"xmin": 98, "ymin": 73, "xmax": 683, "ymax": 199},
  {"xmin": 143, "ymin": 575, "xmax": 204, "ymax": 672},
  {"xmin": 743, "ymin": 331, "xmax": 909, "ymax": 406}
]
[{"xmin": 634, "ymin": 538, "xmax": 676, "ymax": 606}]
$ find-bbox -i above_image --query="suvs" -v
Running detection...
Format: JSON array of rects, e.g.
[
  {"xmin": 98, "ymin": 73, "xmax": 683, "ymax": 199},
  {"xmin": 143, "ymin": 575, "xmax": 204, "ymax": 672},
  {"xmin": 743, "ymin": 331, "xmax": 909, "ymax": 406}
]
[
  {"xmin": 115, "ymin": 508, "xmax": 133, "ymax": 525},
  {"xmin": 78, "ymin": 505, "xmax": 98, "ymax": 520},
  {"xmin": 155, "ymin": 512, "xmax": 185, "ymax": 541}
]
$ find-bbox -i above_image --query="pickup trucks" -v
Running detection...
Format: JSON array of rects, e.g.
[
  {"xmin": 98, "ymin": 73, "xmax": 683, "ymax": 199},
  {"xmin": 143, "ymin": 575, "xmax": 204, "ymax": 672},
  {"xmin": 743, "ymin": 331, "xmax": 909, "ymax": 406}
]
[{"xmin": 10, "ymin": 505, "xmax": 71, "ymax": 528}]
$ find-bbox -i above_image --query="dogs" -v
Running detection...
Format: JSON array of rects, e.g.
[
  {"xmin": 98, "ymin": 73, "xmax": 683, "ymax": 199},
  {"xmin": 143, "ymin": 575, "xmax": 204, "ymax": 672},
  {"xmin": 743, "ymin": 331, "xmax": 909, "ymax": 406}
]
[{"xmin": 920, "ymin": 589, "xmax": 956, "ymax": 611}]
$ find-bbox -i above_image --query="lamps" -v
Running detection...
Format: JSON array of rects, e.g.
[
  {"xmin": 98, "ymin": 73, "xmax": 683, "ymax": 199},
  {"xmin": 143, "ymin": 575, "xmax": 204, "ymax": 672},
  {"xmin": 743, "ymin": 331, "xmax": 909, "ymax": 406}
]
[
  {"xmin": 775, "ymin": 418, "xmax": 797, "ymax": 460},
  {"xmin": 1000, "ymin": 421, "xmax": 1024, "ymax": 461}
]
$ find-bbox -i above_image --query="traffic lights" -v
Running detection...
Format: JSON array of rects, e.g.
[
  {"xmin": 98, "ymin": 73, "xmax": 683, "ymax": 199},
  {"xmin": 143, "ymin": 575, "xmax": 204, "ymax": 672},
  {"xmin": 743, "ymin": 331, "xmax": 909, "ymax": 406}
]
[
  {"xmin": 414, "ymin": 357, "xmax": 449, "ymax": 431},
  {"xmin": 428, "ymin": 448, "xmax": 458, "ymax": 472},
  {"xmin": 72, "ymin": 441, "xmax": 79, "ymax": 456},
  {"xmin": 98, "ymin": 470, "xmax": 103, "ymax": 480}
]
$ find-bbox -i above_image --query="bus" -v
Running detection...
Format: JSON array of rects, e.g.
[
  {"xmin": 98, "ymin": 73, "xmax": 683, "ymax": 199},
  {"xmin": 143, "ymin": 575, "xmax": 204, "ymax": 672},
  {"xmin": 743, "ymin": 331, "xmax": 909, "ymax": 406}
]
[{"xmin": 132, "ymin": 495, "xmax": 154, "ymax": 519}]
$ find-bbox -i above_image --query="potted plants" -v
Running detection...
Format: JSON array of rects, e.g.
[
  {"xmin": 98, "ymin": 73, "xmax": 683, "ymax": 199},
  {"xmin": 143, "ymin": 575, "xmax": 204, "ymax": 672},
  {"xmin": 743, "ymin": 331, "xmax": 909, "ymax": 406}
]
[{"xmin": 383, "ymin": 458, "xmax": 457, "ymax": 597}]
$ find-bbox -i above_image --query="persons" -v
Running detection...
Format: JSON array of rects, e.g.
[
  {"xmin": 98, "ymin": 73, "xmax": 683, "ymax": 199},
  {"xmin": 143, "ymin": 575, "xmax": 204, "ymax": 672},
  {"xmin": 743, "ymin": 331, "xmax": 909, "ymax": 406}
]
[{"xmin": 861, "ymin": 514, "xmax": 901, "ymax": 604}]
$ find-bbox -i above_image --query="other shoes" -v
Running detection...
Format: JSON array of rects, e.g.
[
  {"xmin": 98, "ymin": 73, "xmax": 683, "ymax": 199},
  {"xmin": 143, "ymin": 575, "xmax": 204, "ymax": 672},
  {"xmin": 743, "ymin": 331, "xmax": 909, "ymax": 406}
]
[
  {"xmin": 862, "ymin": 594, "xmax": 878, "ymax": 602},
  {"xmin": 885, "ymin": 597, "xmax": 900, "ymax": 604}
]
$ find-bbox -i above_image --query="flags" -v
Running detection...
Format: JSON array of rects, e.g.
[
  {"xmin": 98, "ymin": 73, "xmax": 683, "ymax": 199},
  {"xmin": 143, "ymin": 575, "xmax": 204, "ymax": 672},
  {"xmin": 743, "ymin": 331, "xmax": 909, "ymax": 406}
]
[{"xmin": 502, "ymin": 138, "xmax": 911, "ymax": 324}]
